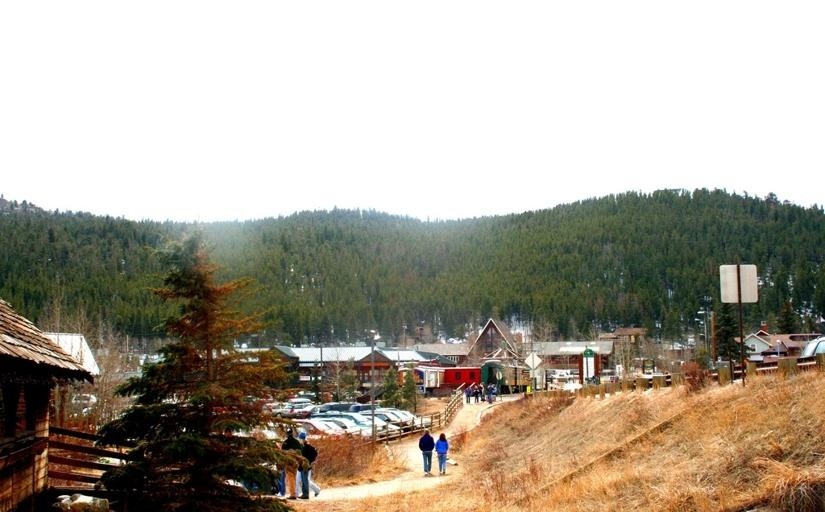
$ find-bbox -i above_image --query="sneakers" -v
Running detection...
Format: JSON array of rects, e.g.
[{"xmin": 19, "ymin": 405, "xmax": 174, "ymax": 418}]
[
  {"xmin": 275, "ymin": 489, "xmax": 319, "ymax": 499},
  {"xmin": 425, "ymin": 471, "xmax": 446, "ymax": 476}
]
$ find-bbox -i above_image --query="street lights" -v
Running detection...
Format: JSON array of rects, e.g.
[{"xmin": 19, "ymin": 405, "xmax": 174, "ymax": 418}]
[{"xmin": 776, "ymin": 340, "xmax": 780, "ymax": 357}]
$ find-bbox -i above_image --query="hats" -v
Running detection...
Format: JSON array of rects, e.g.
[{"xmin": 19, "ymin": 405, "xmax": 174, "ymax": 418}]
[{"xmin": 298, "ymin": 431, "xmax": 306, "ymax": 439}]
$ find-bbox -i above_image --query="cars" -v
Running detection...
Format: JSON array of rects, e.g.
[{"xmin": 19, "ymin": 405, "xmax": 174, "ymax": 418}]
[
  {"xmin": 800, "ymin": 337, "xmax": 825, "ymax": 357},
  {"xmin": 263, "ymin": 398, "xmax": 430, "ymax": 441},
  {"xmin": 72, "ymin": 393, "xmax": 97, "ymax": 407}
]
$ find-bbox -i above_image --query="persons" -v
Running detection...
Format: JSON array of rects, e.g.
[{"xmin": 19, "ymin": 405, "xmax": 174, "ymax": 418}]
[
  {"xmin": 418, "ymin": 428, "xmax": 435, "ymax": 475},
  {"xmin": 465, "ymin": 383, "xmax": 495, "ymax": 403},
  {"xmin": 435, "ymin": 433, "xmax": 448, "ymax": 473},
  {"xmin": 242, "ymin": 431, "xmax": 321, "ymax": 497}
]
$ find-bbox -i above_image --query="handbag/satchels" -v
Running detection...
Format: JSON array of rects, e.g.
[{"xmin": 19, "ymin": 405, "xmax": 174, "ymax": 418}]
[{"xmin": 298, "ymin": 444, "xmax": 317, "ymax": 472}]
